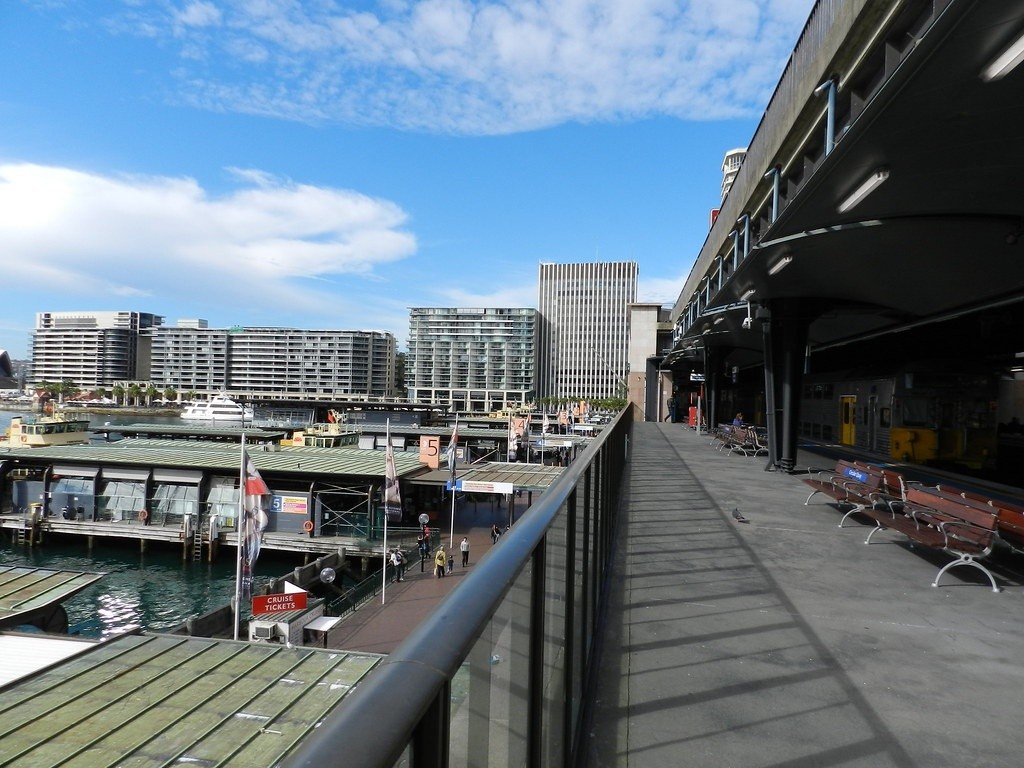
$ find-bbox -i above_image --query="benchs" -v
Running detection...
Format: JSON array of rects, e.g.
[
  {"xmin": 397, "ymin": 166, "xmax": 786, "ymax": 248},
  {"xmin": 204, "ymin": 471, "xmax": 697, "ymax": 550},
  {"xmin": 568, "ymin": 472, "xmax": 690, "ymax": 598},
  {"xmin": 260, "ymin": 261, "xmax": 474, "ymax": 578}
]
[
  {"xmin": 709, "ymin": 421, "xmax": 770, "ymax": 458},
  {"xmin": 801, "ymin": 458, "xmax": 911, "ymax": 529},
  {"xmin": 681, "ymin": 416, "xmax": 707, "ymax": 432},
  {"xmin": 860, "ymin": 481, "xmax": 1023, "ymax": 594}
]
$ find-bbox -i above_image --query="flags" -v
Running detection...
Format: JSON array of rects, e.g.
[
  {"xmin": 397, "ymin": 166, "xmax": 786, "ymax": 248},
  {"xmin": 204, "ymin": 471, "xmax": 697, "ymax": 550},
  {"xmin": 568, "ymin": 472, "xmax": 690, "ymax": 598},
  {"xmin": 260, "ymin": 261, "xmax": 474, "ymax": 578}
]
[
  {"xmin": 509, "ymin": 416, "xmax": 519, "ymax": 459},
  {"xmin": 521, "ymin": 412, "xmax": 530, "ymax": 448},
  {"xmin": 242, "ymin": 450, "xmax": 272, "ymax": 602},
  {"xmin": 544, "ymin": 406, "xmax": 549, "ymax": 433},
  {"xmin": 386, "ymin": 421, "xmax": 403, "ymax": 522},
  {"xmin": 447, "ymin": 419, "xmax": 458, "ymax": 486}
]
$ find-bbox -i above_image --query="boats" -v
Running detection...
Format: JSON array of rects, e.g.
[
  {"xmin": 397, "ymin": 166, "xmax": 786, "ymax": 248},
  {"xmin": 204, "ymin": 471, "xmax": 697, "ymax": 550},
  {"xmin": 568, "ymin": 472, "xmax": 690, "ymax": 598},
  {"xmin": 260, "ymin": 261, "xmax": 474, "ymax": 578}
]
[
  {"xmin": 283, "ymin": 412, "xmax": 360, "ymax": 450},
  {"xmin": 180, "ymin": 390, "xmax": 254, "ymax": 422},
  {"xmin": 10, "ymin": 400, "xmax": 90, "ymax": 447}
]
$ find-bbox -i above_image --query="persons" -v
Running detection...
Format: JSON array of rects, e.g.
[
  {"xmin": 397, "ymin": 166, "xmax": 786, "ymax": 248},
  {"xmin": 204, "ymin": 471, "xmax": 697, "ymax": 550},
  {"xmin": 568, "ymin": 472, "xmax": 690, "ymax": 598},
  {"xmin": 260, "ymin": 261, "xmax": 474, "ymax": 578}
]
[
  {"xmin": 434, "ymin": 547, "xmax": 446, "ymax": 578},
  {"xmin": 419, "ymin": 533, "xmax": 431, "ymax": 558},
  {"xmin": 388, "ymin": 548, "xmax": 408, "ymax": 581},
  {"xmin": 461, "ymin": 537, "xmax": 469, "ymax": 566},
  {"xmin": 491, "ymin": 524, "xmax": 501, "ymax": 544},
  {"xmin": 733, "ymin": 413, "xmax": 744, "ymax": 427},
  {"xmin": 502, "ymin": 525, "xmax": 510, "ymax": 535},
  {"xmin": 448, "ymin": 556, "xmax": 454, "ymax": 574},
  {"xmin": 664, "ymin": 394, "xmax": 678, "ymax": 423}
]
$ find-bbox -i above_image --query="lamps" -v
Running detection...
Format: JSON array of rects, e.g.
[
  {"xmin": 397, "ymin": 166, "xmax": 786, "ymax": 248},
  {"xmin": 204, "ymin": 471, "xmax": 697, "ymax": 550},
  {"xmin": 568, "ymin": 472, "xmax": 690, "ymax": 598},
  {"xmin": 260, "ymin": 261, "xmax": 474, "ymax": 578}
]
[
  {"xmin": 739, "ymin": 286, "xmax": 756, "ymax": 302},
  {"xmin": 766, "ymin": 253, "xmax": 793, "ymax": 277},
  {"xmin": 976, "ymin": 28, "xmax": 1024, "ymax": 89},
  {"xmin": 834, "ymin": 164, "xmax": 891, "ymax": 216}
]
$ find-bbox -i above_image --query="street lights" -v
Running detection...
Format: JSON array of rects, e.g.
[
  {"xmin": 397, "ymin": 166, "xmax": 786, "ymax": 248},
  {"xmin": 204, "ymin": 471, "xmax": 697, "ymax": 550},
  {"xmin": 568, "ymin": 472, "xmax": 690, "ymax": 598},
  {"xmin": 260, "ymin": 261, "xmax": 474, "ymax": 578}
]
[
  {"xmin": 319, "ymin": 567, "xmax": 336, "ymax": 649},
  {"xmin": 419, "ymin": 514, "xmax": 429, "ymax": 572}
]
